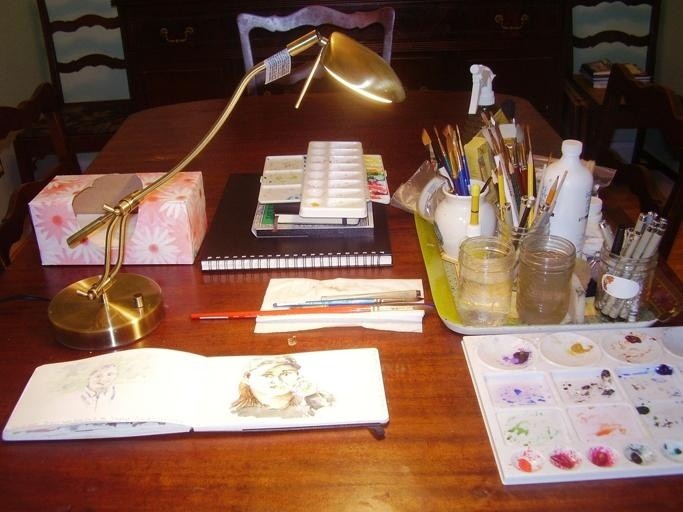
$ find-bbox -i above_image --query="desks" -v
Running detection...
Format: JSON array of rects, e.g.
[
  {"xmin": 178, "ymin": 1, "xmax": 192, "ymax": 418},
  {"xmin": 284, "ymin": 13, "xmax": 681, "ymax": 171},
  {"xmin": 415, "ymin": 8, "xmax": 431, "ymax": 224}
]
[{"xmin": 0, "ymin": 92, "xmax": 680, "ymax": 512}]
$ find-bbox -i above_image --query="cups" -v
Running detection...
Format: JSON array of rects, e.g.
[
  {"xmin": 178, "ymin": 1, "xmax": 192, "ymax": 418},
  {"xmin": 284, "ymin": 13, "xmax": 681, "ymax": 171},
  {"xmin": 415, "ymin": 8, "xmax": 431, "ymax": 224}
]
[
  {"xmin": 455, "ymin": 235, "xmax": 515, "ymax": 326},
  {"xmin": 515, "ymin": 234, "xmax": 577, "ymax": 325},
  {"xmin": 594, "ymin": 239, "xmax": 658, "ymax": 321}
]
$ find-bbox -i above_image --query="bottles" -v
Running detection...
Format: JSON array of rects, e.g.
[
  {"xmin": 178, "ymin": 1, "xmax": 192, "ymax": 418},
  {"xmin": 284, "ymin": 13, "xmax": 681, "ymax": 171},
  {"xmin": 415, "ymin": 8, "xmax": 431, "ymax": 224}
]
[{"xmin": 542, "ymin": 139, "xmax": 593, "ymax": 258}]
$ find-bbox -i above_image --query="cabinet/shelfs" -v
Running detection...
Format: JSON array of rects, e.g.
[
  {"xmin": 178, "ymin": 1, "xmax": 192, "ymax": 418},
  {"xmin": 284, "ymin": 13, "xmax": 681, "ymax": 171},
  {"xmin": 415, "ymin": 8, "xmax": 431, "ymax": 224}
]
[{"xmin": 112, "ymin": 2, "xmax": 571, "ymax": 109}]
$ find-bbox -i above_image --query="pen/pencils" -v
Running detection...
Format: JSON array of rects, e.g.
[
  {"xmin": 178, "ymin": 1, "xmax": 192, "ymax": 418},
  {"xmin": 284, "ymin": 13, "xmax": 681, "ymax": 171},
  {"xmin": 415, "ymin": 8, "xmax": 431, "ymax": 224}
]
[
  {"xmin": 498, "ymin": 153, "xmax": 567, "ymax": 234},
  {"xmin": 479, "ymin": 167, "xmax": 496, "ymax": 195}
]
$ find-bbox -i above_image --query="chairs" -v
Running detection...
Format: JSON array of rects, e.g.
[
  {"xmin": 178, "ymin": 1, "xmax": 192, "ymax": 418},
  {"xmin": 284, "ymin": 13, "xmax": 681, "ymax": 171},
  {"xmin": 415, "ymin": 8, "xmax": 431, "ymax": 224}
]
[
  {"xmin": 577, "ymin": 62, "xmax": 679, "ymax": 261},
  {"xmin": 0, "ymin": 82, "xmax": 82, "ymax": 269},
  {"xmin": 238, "ymin": 7, "xmax": 394, "ymax": 96}
]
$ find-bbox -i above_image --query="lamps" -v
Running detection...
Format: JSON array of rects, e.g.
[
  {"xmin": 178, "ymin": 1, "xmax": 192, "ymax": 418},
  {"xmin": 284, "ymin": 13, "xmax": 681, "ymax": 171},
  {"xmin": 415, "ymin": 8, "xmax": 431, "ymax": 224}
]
[{"xmin": 47, "ymin": 33, "xmax": 405, "ymax": 350}]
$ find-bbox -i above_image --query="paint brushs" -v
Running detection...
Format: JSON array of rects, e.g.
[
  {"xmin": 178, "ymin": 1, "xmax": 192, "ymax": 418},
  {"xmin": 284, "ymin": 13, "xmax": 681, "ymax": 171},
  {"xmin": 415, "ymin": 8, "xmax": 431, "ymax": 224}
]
[
  {"xmin": 191, "ymin": 290, "xmax": 435, "ymax": 323},
  {"xmin": 480, "ymin": 107, "xmax": 526, "ymax": 169},
  {"xmin": 596, "ymin": 210, "xmax": 667, "ymax": 322},
  {"xmin": 420, "ymin": 122, "xmax": 471, "ymax": 198}
]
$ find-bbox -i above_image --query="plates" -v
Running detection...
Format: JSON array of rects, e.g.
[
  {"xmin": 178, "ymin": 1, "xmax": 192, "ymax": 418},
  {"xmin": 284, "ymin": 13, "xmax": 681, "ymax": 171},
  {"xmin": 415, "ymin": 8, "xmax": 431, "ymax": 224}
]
[{"xmin": 412, "ymin": 211, "xmax": 659, "ymax": 336}]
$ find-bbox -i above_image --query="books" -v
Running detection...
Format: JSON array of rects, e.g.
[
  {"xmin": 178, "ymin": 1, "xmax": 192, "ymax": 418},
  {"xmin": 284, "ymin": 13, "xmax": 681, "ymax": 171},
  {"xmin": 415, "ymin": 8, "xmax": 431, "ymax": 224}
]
[
  {"xmin": 251, "ymin": 202, "xmax": 376, "ymax": 237},
  {"xmin": 199, "ymin": 173, "xmax": 392, "ymax": 273},
  {"xmin": 1, "ymin": 347, "xmax": 390, "ymax": 442},
  {"xmin": 582, "ymin": 58, "xmax": 649, "ymax": 89}
]
